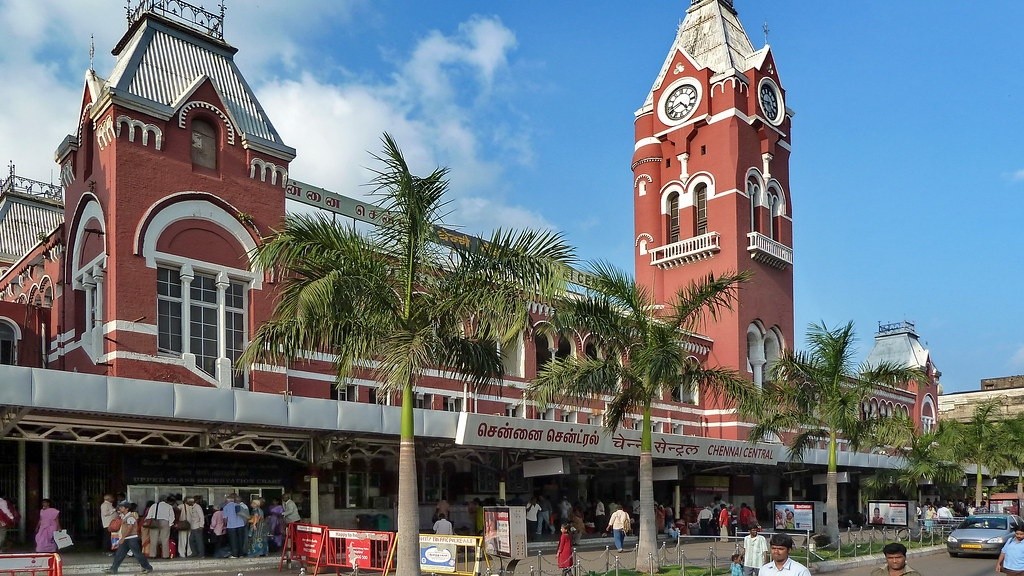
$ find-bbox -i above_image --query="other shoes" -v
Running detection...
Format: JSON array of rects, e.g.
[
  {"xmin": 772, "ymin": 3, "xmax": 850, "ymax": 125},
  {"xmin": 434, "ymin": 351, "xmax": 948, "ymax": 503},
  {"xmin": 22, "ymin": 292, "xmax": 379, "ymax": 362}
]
[
  {"xmin": 140, "ymin": 566, "xmax": 154, "ymax": 574},
  {"xmin": 536, "ymin": 532, "xmax": 541, "ymax": 534},
  {"xmin": 228, "ymin": 555, "xmax": 237, "ymax": 558},
  {"xmin": 551, "ymin": 529, "xmax": 556, "ymax": 534},
  {"xmin": 618, "ymin": 548, "xmax": 623, "ymax": 553},
  {"xmin": 103, "ymin": 568, "xmax": 118, "ymax": 573}
]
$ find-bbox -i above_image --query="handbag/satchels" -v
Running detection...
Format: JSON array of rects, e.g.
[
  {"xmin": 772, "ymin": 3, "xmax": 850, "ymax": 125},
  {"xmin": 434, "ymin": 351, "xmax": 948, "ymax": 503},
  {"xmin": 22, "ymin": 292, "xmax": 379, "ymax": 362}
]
[
  {"xmin": 721, "ymin": 527, "xmax": 728, "ymax": 536},
  {"xmin": 143, "ymin": 518, "xmax": 158, "ymax": 528},
  {"xmin": 108, "ymin": 516, "xmax": 122, "ymax": 532},
  {"xmin": 178, "ymin": 520, "xmax": 191, "ymax": 530},
  {"xmin": 624, "ymin": 512, "xmax": 632, "ymax": 532},
  {"xmin": 205, "ymin": 531, "xmax": 216, "ymax": 543},
  {"xmin": 111, "ymin": 537, "xmax": 120, "ymax": 550},
  {"xmin": 432, "ymin": 509, "xmax": 439, "ymax": 523},
  {"xmin": 550, "ymin": 514, "xmax": 554, "ymax": 525},
  {"xmin": 999, "ymin": 557, "xmax": 1005, "ymax": 573},
  {"xmin": 0, "ymin": 501, "xmax": 21, "ymax": 527},
  {"xmin": 53, "ymin": 529, "xmax": 74, "ymax": 549}
]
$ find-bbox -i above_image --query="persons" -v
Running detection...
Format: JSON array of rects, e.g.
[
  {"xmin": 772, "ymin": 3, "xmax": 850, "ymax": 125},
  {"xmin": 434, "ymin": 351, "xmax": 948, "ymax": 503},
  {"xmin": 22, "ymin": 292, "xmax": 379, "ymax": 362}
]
[
  {"xmin": 606, "ymin": 505, "xmax": 631, "ymax": 552},
  {"xmin": 432, "ymin": 499, "xmax": 453, "ymax": 535},
  {"xmin": 654, "ymin": 500, "xmax": 757, "ymax": 540},
  {"xmin": 526, "ymin": 494, "xmax": 608, "ymax": 537},
  {"xmin": 917, "ymin": 497, "xmax": 989, "ymax": 536},
  {"xmin": 0, "ymin": 497, "xmax": 21, "ymax": 552},
  {"xmin": 872, "ymin": 508, "xmax": 885, "ymax": 524},
  {"xmin": 719, "ymin": 503, "xmax": 729, "ymax": 542},
  {"xmin": 609, "ymin": 496, "xmax": 640, "ymax": 536},
  {"xmin": 871, "ymin": 543, "xmax": 921, "ymax": 576},
  {"xmin": 729, "ymin": 554, "xmax": 744, "ymax": 576},
  {"xmin": 466, "ymin": 496, "xmax": 508, "ymax": 537},
  {"xmin": 775, "ymin": 508, "xmax": 795, "ymax": 529},
  {"xmin": 758, "ymin": 533, "xmax": 811, "ymax": 576},
  {"xmin": 99, "ymin": 491, "xmax": 300, "ymax": 572},
  {"xmin": 995, "ymin": 526, "xmax": 1024, "ymax": 576},
  {"xmin": 554, "ymin": 523, "xmax": 576, "ymax": 576},
  {"xmin": 739, "ymin": 524, "xmax": 769, "ymax": 576},
  {"xmin": 1009, "ymin": 501, "xmax": 1018, "ymax": 515},
  {"xmin": 34, "ymin": 499, "xmax": 61, "ymax": 552}
]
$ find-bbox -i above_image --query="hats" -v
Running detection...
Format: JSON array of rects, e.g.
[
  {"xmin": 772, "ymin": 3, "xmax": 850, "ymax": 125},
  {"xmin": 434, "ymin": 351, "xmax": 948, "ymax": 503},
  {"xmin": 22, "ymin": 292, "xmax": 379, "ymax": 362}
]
[
  {"xmin": 118, "ymin": 500, "xmax": 129, "ymax": 508},
  {"xmin": 771, "ymin": 534, "xmax": 793, "ymax": 549},
  {"xmin": 213, "ymin": 505, "xmax": 221, "ymax": 510}
]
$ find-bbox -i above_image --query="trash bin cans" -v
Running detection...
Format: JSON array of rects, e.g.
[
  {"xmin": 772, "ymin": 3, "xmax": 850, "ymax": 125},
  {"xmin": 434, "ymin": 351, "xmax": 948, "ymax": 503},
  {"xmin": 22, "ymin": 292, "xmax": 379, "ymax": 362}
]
[
  {"xmin": 375, "ymin": 514, "xmax": 390, "ymax": 551},
  {"xmin": 356, "ymin": 514, "xmax": 375, "ymax": 551}
]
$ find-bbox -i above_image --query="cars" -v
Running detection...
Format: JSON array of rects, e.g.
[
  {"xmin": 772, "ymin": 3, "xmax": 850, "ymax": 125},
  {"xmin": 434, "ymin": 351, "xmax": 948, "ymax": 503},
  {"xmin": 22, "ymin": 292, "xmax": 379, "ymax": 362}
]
[{"xmin": 946, "ymin": 513, "xmax": 1024, "ymax": 558}]
